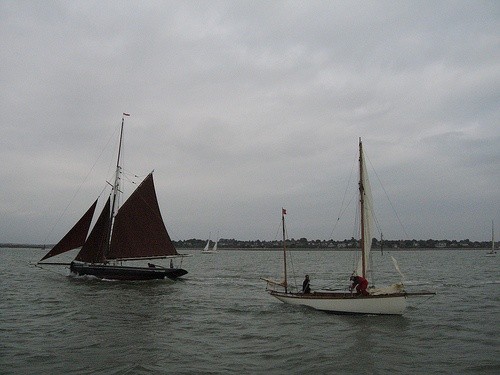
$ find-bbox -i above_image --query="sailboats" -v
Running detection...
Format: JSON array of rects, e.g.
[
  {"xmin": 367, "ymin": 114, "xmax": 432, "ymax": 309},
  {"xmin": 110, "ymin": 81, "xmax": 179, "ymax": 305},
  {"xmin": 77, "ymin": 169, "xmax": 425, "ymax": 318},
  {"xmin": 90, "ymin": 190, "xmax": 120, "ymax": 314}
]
[
  {"xmin": 259, "ymin": 136, "xmax": 436, "ymax": 315},
  {"xmin": 483, "ymin": 220, "xmax": 498, "ymax": 257},
  {"xmin": 201, "ymin": 232, "xmax": 220, "ymax": 254},
  {"xmin": 25, "ymin": 112, "xmax": 194, "ymax": 280}
]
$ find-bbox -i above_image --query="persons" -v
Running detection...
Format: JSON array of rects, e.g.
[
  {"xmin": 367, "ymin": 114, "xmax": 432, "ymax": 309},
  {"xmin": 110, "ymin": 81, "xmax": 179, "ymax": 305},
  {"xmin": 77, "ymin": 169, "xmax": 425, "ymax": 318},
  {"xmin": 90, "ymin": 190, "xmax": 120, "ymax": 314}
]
[
  {"xmin": 303, "ymin": 275, "xmax": 311, "ymax": 293},
  {"xmin": 148, "ymin": 263, "xmax": 155, "ymax": 268},
  {"xmin": 349, "ymin": 276, "xmax": 368, "ymax": 295},
  {"xmin": 170, "ymin": 259, "xmax": 173, "ymax": 268}
]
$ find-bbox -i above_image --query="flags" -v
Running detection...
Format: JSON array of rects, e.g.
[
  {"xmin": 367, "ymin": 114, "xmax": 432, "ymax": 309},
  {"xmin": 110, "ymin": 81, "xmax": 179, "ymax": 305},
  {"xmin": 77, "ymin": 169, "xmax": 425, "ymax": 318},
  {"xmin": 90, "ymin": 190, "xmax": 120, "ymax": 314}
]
[
  {"xmin": 283, "ymin": 209, "xmax": 286, "ymax": 214},
  {"xmin": 123, "ymin": 113, "xmax": 130, "ymax": 116}
]
